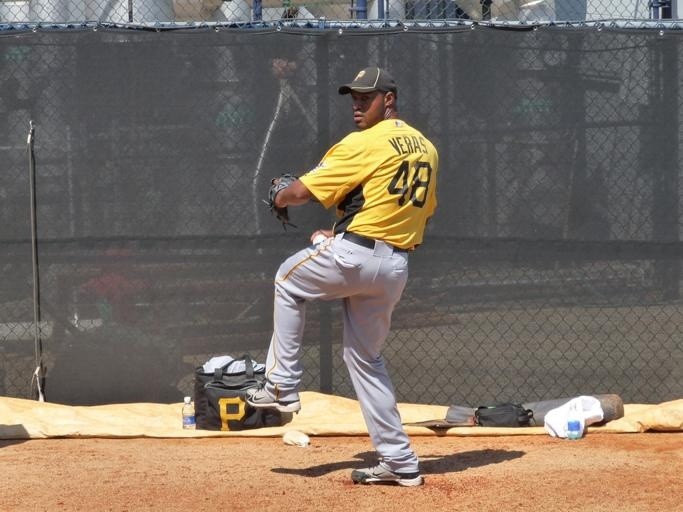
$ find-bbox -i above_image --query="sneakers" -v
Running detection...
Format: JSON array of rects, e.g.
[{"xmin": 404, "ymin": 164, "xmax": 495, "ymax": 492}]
[
  {"xmin": 245, "ymin": 380, "xmax": 301, "ymax": 412},
  {"xmin": 350, "ymin": 464, "xmax": 422, "ymax": 486}
]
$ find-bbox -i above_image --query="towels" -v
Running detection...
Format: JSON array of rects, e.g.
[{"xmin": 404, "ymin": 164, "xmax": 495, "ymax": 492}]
[{"xmin": 544, "ymin": 396, "xmax": 602, "ymax": 437}]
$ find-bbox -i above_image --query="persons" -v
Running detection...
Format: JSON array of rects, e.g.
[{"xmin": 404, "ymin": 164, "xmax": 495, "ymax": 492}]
[{"xmin": 245, "ymin": 68, "xmax": 438, "ymax": 486}]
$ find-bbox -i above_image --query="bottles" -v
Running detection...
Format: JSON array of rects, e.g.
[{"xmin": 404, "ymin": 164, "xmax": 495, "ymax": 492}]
[
  {"xmin": 566, "ymin": 402, "xmax": 581, "ymax": 439},
  {"xmin": 180, "ymin": 396, "xmax": 196, "ymax": 429}
]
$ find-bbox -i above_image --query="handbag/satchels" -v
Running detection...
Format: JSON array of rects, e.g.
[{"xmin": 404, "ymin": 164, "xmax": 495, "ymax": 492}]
[
  {"xmin": 473, "ymin": 401, "xmax": 533, "ymax": 426},
  {"xmin": 193, "ymin": 354, "xmax": 293, "ymax": 431}
]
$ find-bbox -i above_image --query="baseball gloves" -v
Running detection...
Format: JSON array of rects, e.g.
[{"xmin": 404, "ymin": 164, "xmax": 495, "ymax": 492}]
[{"xmin": 267, "ymin": 171, "xmax": 301, "ymax": 229}]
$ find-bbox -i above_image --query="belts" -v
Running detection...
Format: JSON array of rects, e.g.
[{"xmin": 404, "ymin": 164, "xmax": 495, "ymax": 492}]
[{"xmin": 343, "ymin": 231, "xmax": 407, "ymax": 252}]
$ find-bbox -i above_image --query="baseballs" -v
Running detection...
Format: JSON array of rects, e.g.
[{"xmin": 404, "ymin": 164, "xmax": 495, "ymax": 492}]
[{"xmin": 312, "ymin": 234, "xmax": 326, "ymax": 246}]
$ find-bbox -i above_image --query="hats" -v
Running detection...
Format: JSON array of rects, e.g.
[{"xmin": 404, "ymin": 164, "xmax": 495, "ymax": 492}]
[{"xmin": 338, "ymin": 67, "xmax": 397, "ymax": 96}]
